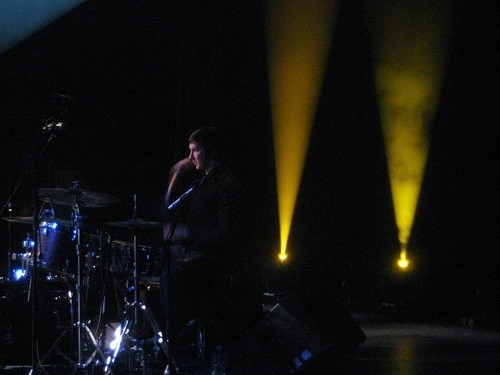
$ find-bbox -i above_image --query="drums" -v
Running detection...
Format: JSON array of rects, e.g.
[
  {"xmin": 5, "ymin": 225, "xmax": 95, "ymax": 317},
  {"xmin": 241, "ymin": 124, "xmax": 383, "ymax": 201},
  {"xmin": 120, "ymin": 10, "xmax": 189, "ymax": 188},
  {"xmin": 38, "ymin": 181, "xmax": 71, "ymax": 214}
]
[
  {"xmin": 11, "ymin": 250, "xmax": 38, "ymax": 289},
  {"xmin": 37, "ymin": 218, "xmax": 82, "ymax": 276},
  {"xmin": 109, "ymin": 239, "xmax": 154, "ymax": 277}
]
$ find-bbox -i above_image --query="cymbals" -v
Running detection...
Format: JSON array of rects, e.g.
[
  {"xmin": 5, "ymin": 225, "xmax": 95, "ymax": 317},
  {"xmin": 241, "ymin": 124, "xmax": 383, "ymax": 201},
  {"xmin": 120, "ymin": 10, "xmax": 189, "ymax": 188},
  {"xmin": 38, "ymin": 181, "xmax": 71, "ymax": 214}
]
[
  {"xmin": 38, "ymin": 187, "xmax": 111, "ymax": 208},
  {"xmin": 2, "ymin": 217, "xmax": 35, "ymax": 224},
  {"xmin": 108, "ymin": 218, "xmax": 161, "ymax": 231}
]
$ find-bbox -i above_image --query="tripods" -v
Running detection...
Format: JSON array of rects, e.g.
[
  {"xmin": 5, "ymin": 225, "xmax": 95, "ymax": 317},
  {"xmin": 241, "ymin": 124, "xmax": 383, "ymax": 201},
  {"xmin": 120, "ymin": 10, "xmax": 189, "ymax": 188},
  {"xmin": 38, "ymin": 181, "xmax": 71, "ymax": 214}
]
[{"xmin": 0, "ymin": 124, "xmax": 70, "ymax": 375}]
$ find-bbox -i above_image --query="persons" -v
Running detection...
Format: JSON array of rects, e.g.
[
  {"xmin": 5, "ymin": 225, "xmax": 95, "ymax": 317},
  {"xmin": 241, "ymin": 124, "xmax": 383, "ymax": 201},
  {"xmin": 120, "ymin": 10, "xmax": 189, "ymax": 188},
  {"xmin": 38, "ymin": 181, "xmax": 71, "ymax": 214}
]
[{"xmin": 160, "ymin": 125, "xmax": 243, "ymax": 306}]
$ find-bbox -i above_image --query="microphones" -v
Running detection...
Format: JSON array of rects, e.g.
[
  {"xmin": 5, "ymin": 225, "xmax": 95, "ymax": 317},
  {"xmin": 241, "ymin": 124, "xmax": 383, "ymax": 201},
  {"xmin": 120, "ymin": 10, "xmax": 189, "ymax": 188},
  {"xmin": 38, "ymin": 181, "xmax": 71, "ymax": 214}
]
[{"xmin": 168, "ymin": 186, "xmax": 194, "ymax": 211}]
